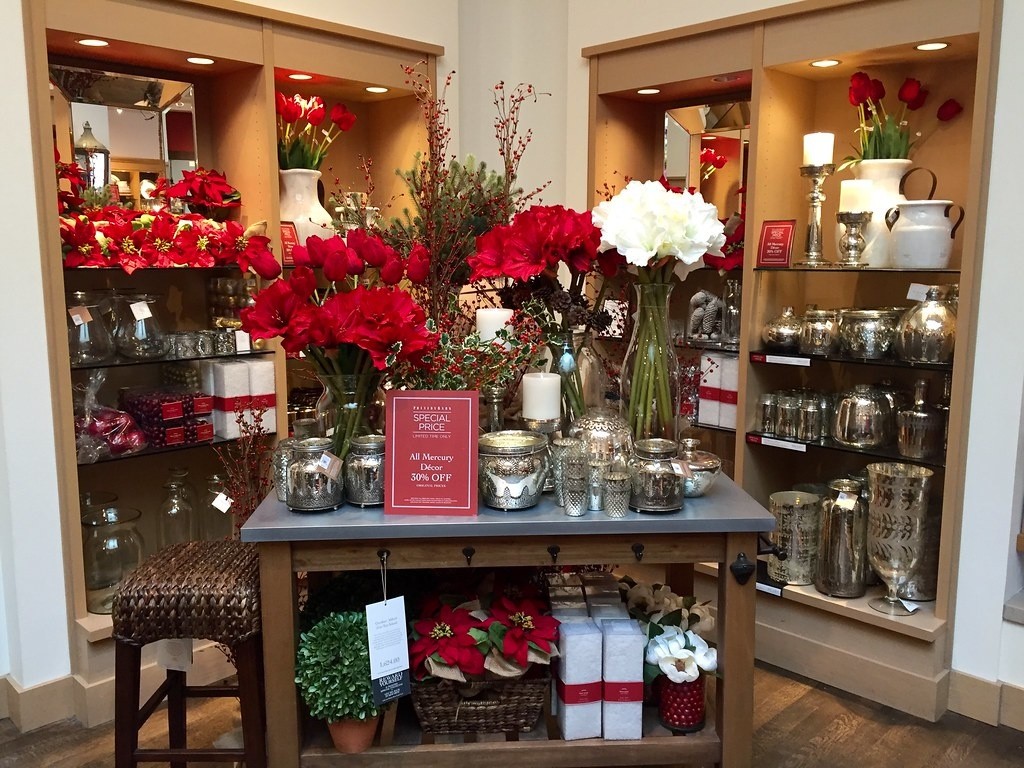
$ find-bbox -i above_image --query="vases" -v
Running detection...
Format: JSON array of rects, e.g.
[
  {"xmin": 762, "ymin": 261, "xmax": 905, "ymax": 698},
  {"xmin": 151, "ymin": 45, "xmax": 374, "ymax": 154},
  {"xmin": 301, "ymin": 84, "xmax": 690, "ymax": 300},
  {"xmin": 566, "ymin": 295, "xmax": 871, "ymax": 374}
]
[
  {"xmin": 311, "ymin": 370, "xmax": 376, "ymax": 462},
  {"xmin": 880, "ymin": 198, "xmax": 966, "ymax": 269},
  {"xmin": 833, "ymin": 157, "xmax": 914, "ymax": 255},
  {"xmin": 275, "ymin": 167, "xmax": 335, "ymax": 248},
  {"xmin": 618, "ymin": 281, "xmax": 682, "ymax": 439},
  {"xmin": 658, "ymin": 668, "xmax": 705, "ymax": 734},
  {"xmin": 525, "ymin": 326, "xmax": 603, "ymax": 415}
]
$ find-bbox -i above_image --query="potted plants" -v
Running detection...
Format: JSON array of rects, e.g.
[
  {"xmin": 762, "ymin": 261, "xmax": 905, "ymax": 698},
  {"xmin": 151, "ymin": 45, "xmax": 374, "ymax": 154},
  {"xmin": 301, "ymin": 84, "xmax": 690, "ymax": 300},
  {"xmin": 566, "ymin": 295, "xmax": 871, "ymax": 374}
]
[{"xmin": 292, "ymin": 610, "xmax": 400, "ymax": 757}]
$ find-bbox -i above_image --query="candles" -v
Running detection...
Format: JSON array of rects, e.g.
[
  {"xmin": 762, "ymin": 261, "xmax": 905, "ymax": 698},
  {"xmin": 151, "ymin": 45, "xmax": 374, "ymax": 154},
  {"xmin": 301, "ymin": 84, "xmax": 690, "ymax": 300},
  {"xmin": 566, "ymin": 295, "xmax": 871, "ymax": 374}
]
[
  {"xmin": 519, "ymin": 367, "xmax": 561, "ymax": 421},
  {"xmin": 802, "ymin": 131, "xmax": 834, "ymax": 166},
  {"xmin": 472, "ymin": 301, "xmax": 512, "ymax": 354}
]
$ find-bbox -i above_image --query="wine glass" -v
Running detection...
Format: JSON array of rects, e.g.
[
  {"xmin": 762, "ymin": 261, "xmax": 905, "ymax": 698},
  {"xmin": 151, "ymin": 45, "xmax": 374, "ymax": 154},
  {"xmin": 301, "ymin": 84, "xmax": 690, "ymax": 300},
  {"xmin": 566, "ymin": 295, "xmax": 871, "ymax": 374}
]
[{"xmin": 866, "ymin": 461, "xmax": 934, "ymax": 618}]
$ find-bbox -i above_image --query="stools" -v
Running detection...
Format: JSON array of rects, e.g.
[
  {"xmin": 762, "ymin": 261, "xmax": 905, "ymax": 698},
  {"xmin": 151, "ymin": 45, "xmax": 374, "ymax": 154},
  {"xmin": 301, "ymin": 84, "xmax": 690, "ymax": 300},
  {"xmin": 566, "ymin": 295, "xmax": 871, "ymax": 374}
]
[{"xmin": 111, "ymin": 536, "xmax": 267, "ymax": 767}]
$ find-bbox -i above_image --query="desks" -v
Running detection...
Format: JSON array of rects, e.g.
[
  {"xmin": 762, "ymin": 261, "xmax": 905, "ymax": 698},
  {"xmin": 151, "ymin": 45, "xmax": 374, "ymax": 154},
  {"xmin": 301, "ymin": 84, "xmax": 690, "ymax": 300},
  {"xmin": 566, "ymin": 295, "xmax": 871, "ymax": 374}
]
[{"xmin": 239, "ymin": 468, "xmax": 775, "ymax": 768}]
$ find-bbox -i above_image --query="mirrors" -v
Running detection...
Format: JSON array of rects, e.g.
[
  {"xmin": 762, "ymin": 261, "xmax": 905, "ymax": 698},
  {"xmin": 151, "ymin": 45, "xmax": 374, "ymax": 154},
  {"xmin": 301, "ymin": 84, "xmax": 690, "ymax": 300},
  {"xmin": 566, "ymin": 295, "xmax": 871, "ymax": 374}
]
[
  {"xmin": 48, "ymin": 62, "xmax": 197, "ymax": 216},
  {"xmin": 660, "ymin": 99, "xmax": 750, "ymax": 226}
]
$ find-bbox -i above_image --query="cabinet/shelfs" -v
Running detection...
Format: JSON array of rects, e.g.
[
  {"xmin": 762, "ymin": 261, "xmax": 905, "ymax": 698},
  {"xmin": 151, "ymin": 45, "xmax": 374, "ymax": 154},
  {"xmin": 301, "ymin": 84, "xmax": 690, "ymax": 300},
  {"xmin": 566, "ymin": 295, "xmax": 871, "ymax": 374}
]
[
  {"xmin": 21, "ymin": 0, "xmax": 444, "ymax": 636},
  {"xmin": 582, "ymin": 0, "xmax": 996, "ymax": 722}
]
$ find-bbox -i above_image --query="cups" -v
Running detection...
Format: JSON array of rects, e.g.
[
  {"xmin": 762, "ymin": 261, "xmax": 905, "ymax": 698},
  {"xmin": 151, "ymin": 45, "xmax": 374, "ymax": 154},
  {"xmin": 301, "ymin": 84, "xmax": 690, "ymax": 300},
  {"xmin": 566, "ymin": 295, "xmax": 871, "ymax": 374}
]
[
  {"xmin": 552, "ymin": 438, "xmax": 632, "ymax": 517},
  {"xmin": 898, "ymin": 479, "xmax": 942, "ymax": 602},
  {"xmin": 767, "ymin": 481, "xmax": 826, "ymax": 586}
]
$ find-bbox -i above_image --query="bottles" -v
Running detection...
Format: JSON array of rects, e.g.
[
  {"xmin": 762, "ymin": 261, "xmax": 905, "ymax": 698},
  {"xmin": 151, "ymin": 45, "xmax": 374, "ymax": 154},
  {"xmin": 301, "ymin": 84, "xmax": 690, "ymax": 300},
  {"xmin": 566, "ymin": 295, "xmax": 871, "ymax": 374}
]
[
  {"xmin": 761, "ymin": 281, "xmax": 959, "ymax": 366},
  {"xmin": 157, "ymin": 464, "xmax": 234, "ymax": 551},
  {"xmin": 884, "ymin": 200, "xmax": 964, "ymax": 270},
  {"xmin": 754, "ymin": 371, "xmax": 950, "ymax": 461},
  {"xmin": 814, "ymin": 469, "xmax": 882, "ymax": 599},
  {"xmin": 720, "ymin": 280, "xmax": 742, "ymax": 350},
  {"xmin": 678, "ymin": 439, "xmax": 723, "ymax": 497},
  {"xmin": 65, "ymin": 286, "xmax": 172, "ymax": 369},
  {"xmin": 269, "ymin": 417, "xmax": 387, "ymax": 513},
  {"xmin": 565, "ymin": 406, "xmax": 636, "ymax": 463},
  {"xmin": 477, "ymin": 430, "xmax": 554, "ymax": 511},
  {"xmin": 78, "ymin": 491, "xmax": 148, "ymax": 615},
  {"xmin": 626, "ymin": 437, "xmax": 687, "ymax": 513}
]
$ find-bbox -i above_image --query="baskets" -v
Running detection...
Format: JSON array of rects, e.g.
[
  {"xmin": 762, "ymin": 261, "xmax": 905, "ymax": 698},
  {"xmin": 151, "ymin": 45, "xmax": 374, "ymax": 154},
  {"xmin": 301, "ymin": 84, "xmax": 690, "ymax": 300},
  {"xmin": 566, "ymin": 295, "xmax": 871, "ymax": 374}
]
[{"xmin": 406, "ymin": 670, "xmax": 552, "ymax": 734}]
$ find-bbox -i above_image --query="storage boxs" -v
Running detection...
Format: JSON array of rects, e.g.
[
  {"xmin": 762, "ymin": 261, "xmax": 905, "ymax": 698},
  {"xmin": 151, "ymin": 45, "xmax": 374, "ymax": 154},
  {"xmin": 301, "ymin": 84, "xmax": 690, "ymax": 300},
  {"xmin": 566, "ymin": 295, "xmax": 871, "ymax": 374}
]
[
  {"xmin": 107, "ymin": 353, "xmax": 276, "ymax": 449},
  {"xmin": 692, "ymin": 353, "xmax": 738, "ymax": 428},
  {"xmin": 542, "ymin": 569, "xmax": 645, "ymax": 742}
]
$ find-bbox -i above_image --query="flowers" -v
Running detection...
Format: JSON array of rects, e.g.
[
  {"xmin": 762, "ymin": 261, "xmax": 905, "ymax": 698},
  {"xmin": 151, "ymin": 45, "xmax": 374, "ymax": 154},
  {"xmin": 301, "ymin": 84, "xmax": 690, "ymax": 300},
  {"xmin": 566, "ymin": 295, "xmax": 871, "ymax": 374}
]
[
  {"xmin": 587, "ymin": 178, "xmax": 724, "ymax": 439},
  {"xmin": 272, "ymin": 88, "xmax": 357, "ymax": 170},
  {"xmin": 236, "ymin": 230, "xmax": 440, "ymax": 461},
  {"xmin": 619, "ymin": 574, "xmax": 722, "ymax": 682},
  {"xmin": 699, "ymin": 145, "xmax": 728, "ymax": 184},
  {"xmin": 830, "ymin": 69, "xmax": 966, "ymax": 174},
  {"xmin": 408, "ymin": 586, "xmax": 560, "ymax": 681},
  {"xmin": 462, "ymin": 203, "xmax": 628, "ymax": 422},
  {"xmin": 54, "ymin": 144, "xmax": 280, "ymax": 280}
]
